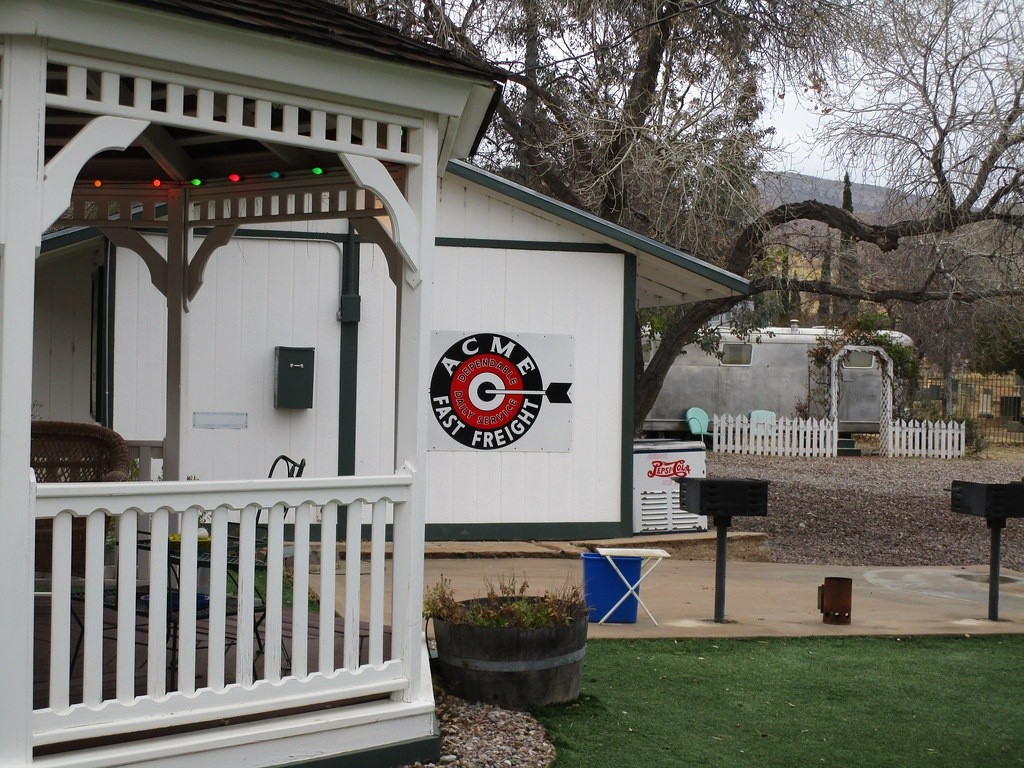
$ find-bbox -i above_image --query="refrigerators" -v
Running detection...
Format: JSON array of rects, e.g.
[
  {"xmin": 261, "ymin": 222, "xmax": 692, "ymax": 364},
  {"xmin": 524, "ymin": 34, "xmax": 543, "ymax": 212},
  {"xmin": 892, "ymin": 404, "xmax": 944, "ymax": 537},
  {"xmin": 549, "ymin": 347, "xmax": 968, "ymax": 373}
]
[{"xmin": 632, "ymin": 438, "xmax": 707, "ymax": 533}]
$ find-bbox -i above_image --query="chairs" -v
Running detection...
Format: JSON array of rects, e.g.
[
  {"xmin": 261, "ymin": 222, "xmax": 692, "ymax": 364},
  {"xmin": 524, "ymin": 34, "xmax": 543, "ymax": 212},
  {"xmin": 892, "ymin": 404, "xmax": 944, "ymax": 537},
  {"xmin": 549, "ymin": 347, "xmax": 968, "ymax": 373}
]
[
  {"xmin": 686, "ymin": 407, "xmax": 721, "ymax": 444},
  {"xmin": 28, "ymin": 421, "xmax": 131, "ymax": 676},
  {"xmin": 146, "ymin": 455, "xmax": 307, "ymax": 693},
  {"xmin": 750, "ymin": 410, "xmax": 775, "ymax": 447}
]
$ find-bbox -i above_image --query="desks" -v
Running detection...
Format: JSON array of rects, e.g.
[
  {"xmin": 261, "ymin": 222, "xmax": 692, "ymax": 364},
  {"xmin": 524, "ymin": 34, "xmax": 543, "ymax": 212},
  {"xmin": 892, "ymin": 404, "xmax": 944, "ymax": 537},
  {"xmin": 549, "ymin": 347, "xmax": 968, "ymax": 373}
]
[
  {"xmin": 70, "ymin": 529, "xmax": 265, "ymax": 693},
  {"xmin": 595, "ymin": 546, "xmax": 671, "ymax": 626}
]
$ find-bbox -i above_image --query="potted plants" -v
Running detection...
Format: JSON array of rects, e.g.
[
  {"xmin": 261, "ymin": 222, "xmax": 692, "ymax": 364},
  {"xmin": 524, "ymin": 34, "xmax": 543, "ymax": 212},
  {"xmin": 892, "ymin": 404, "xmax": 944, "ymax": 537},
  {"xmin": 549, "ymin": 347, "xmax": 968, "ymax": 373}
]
[{"xmin": 421, "ymin": 570, "xmax": 591, "ymax": 713}]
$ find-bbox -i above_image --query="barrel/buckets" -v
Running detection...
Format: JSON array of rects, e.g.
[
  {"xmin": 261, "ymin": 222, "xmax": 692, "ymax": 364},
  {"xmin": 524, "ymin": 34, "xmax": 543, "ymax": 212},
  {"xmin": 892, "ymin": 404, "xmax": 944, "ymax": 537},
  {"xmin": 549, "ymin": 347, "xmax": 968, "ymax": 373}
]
[{"xmin": 581, "ymin": 553, "xmax": 642, "ymax": 623}]
